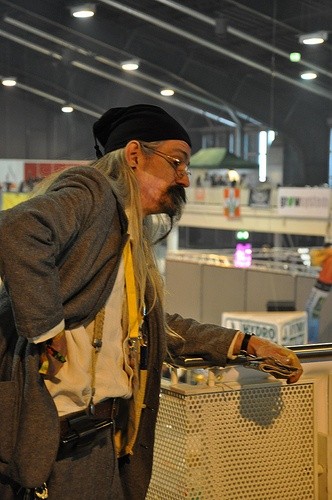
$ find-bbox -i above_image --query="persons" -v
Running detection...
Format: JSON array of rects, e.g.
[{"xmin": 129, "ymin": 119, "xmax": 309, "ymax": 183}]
[
  {"xmin": 195, "ymin": 171, "xmax": 251, "ymax": 188},
  {"xmin": 0, "ymin": 104, "xmax": 304, "ymax": 500}
]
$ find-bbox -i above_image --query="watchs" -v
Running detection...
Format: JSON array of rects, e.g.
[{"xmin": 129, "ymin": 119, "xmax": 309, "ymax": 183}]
[{"xmin": 240, "ymin": 331, "xmax": 256, "ymax": 355}]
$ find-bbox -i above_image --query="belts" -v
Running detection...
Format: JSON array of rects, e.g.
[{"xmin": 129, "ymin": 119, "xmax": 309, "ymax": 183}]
[{"xmin": 59, "ymin": 398, "xmax": 123, "ymax": 453}]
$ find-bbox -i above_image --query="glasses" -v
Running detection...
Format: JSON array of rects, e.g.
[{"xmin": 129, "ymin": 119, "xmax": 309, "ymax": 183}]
[{"xmin": 144, "ymin": 145, "xmax": 192, "ymax": 178}]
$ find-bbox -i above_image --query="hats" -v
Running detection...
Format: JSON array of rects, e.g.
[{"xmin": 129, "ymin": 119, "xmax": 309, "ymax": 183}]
[{"xmin": 92, "ymin": 104, "xmax": 192, "ymax": 159}]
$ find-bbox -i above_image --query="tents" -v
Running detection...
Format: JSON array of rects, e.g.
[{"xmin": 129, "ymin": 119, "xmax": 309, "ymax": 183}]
[{"xmin": 187, "ymin": 147, "xmax": 258, "ymax": 169}]
